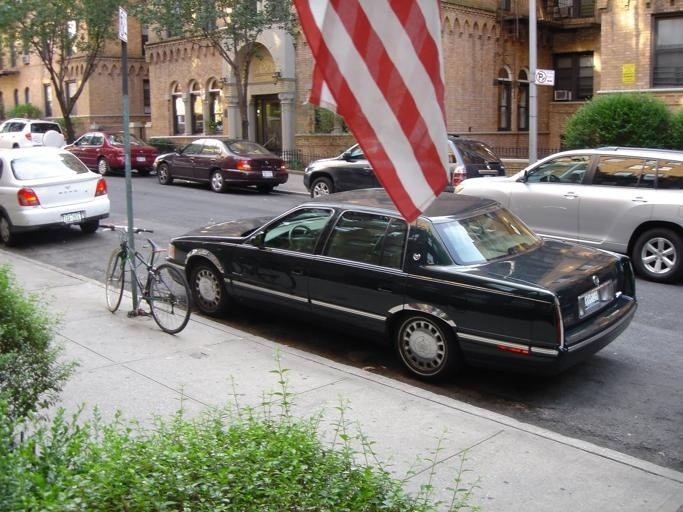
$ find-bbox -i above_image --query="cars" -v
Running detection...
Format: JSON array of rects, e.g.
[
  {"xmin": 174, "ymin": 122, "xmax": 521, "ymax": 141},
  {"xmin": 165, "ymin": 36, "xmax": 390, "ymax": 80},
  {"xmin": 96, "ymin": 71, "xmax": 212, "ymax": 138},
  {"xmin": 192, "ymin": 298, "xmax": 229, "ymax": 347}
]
[
  {"xmin": 152, "ymin": 136, "xmax": 288, "ymax": 193},
  {"xmin": 303, "ymin": 134, "xmax": 506, "ymax": 199},
  {"xmin": 0, "ymin": 146, "xmax": 112, "ymax": 248},
  {"xmin": 453, "ymin": 146, "xmax": 683, "ymax": 283},
  {"xmin": 164, "ymin": 191, "xmax": 639, "ymax": 386},
  {"xmin": 63, "ymin": 130, "xmax": 160, "ymax": 175}
]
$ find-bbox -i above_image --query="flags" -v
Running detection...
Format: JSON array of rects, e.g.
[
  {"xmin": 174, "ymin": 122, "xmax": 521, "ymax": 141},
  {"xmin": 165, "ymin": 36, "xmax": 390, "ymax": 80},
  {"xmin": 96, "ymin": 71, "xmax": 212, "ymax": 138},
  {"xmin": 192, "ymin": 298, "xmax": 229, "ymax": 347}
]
[{"xmin": 292, "ymin": 1, "xmax": 450, "ymax": 226}]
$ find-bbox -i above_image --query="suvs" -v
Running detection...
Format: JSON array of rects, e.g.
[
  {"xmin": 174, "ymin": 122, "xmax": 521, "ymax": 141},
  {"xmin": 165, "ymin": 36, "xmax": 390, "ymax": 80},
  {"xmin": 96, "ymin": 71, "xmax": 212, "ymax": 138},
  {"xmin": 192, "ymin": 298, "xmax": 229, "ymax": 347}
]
[{"xmin": 0, "ymin": 117, "xmax": 66, "ymax": 148}]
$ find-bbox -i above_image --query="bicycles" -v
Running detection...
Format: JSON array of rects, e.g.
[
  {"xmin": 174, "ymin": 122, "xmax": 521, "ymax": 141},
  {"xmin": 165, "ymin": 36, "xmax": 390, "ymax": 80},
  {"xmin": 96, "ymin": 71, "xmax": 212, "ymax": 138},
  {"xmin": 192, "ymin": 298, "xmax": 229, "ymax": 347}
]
[{"xmin": 98, "ymin": 225, "xmax": 193, "ymax": 334}]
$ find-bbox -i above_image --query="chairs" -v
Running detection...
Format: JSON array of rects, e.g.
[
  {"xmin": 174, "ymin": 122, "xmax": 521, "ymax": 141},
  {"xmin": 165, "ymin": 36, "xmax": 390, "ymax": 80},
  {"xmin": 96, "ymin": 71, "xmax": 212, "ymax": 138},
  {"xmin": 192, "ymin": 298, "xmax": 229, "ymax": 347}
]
[{"xmin": 327, "ymin": 226, "xmax": 403, "ymax": 268}]
